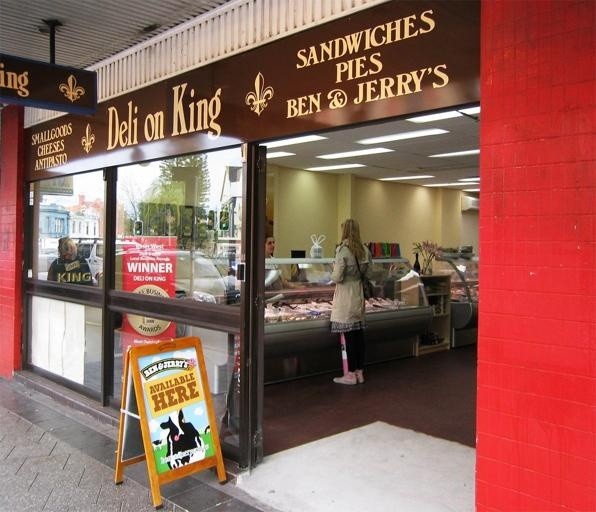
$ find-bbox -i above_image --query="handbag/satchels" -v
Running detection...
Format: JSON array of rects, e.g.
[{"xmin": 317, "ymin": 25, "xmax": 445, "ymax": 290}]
[{"xmin": 362, "ymin": 276, "xmax": 373, "ymax": 298}]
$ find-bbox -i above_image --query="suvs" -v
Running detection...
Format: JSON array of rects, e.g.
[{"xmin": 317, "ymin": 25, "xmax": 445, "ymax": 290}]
[{"xmin": 71, "ymin": 237, "xmax": 143, "ymax": 286}]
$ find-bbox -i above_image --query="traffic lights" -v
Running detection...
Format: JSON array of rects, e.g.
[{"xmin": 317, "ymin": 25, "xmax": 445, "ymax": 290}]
[
  {"xmin": 220, "ymin": 211, "xmax": 229, "ymax": 231},
  {"xmin": 207, "ymin": 210, "xmax": 215, "ymax": 231},
  {"xmin": 134, "ymin": 220, "xmax": 143, "ymax": 236}
]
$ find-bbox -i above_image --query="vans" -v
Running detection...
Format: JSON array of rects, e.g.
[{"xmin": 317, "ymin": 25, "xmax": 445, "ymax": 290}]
[{"xmin": 115, "ymin": 249, "xmax": 241, "ymax": 305}]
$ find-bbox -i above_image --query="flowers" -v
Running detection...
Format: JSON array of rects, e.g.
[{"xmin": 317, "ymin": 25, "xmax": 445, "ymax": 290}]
[{"xmin": 410, "ymin": 240, "xmax": 442, "ymax": 273}]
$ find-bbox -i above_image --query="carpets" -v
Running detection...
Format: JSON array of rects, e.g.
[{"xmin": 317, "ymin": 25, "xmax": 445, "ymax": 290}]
[{"xmin": 229, "ymin": 417, "xmax": 475, "ymax": 511}]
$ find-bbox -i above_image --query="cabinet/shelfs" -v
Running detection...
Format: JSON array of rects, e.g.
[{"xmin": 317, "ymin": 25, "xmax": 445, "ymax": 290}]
[{"xmin": 413, "ymin": 271, "xmax": 452, "ymax": 358}]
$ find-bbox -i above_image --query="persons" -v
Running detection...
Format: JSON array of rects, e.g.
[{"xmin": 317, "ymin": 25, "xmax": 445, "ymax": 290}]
[
  {"xmin": 47, "ymin": 237, "xmax": 94, "ymax": 286},
  {"xmin": 264, "ymin": 235, "xmax": 294, "ymax": 291},
  {"xmin": 331, "ymin": 219, "xmax": 374, "ymax": 385}
]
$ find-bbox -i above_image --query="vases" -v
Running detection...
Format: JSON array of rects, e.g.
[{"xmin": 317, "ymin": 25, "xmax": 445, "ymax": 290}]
[{"xmin": 422, "ymin": 258, "xmax": 433, "ymax": 275}]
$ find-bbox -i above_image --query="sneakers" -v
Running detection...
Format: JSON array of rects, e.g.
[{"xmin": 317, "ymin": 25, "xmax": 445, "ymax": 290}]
[{"xmin": 333, "ymin": 369, "xmax": 364, "ymax": 385}]
